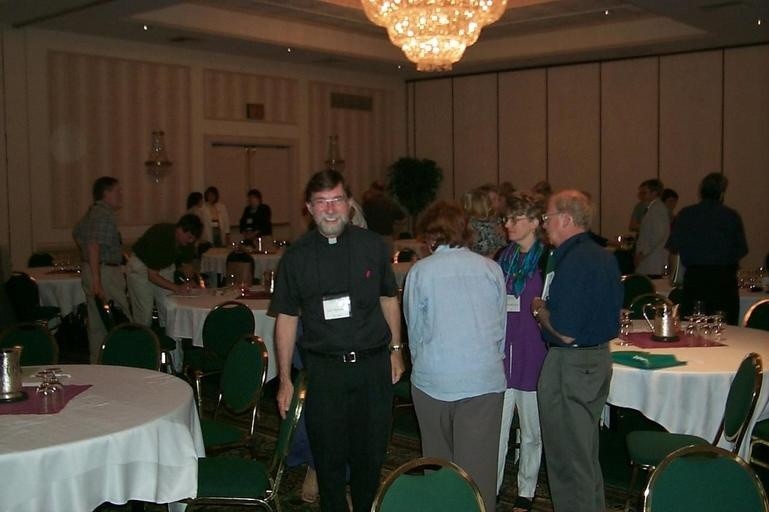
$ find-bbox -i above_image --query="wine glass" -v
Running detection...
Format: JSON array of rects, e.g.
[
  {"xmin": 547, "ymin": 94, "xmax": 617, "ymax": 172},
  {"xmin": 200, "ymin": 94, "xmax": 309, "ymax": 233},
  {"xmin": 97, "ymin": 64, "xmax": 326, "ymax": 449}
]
[
  {"xmin": 683, "ymin": 313, "xmax": 728, "ymax": 345},
  {"xmin": 616, "ymin": 307, "xmax": 634, "ymax": 346},
  {"xmin": 31, "ymin": 365, "xmax": 64, "ymax": 402},
  {"xmin": 735, "ymin": 268, "xmax": 765, "ymax": 291}
]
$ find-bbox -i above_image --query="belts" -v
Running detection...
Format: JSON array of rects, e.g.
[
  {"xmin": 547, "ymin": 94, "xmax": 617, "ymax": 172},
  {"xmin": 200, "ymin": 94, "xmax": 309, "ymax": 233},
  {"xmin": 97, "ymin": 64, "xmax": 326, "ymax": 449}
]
[
  {"xmin": 105, "ymin": 263, "xmax": 119, "ymax": 267},
  {"xmin": 549, "ymin": 342, "xmax": 596, "ymax": 347},
  {"xmin": 312, "ymin": 346, "xmax": 385, "ymax": 363}
]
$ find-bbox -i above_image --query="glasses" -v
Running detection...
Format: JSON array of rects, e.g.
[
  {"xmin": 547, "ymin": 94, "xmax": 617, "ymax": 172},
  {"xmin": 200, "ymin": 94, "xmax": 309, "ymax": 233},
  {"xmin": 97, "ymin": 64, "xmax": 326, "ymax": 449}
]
[
  {"xmin": 540, "ymin": 213, "xmax": 557, "ymax": 223},
  {"xmin": 314, "ymin": 198, "xmax": 344, "ymax": 206},
  {"xmin": 502, "ymin": 216, "xmax": 528, "ymax": 224}
]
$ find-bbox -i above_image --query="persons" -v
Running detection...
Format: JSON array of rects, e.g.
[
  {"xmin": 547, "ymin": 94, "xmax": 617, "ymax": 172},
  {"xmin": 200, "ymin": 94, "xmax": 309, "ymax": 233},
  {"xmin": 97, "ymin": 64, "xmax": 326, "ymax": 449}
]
[
  {"xmin": 496, "ymin": 189, "xmax": 548, "ymax": 512},
  {"xmin": 402, "ymin": 200, "xmax": 507, "ymax": 512},
  {"xmin": 531, "ymin": 188, "xmax": 624, "ymax": 512},
  {"xmin": 266, "ymin": 169, "xmax": 406, "ymax": 512}
]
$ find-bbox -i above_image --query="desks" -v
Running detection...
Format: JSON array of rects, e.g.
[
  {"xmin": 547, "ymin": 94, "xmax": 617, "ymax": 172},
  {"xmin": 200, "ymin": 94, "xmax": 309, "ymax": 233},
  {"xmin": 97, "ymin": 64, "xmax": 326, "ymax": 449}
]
[
  {"xmin": 608, "ymin": 319, "xmax": 767, "ymax": 462},
  {"xmin": 737, "ymin": 278, "xmax": 767, "ymax": 326},
  {"xmin": 12, "ymin": 266, "xmax": 89, "ymax": 316},
  {"xmin": 156, "ymin": 287, "xmax": 276, "ymax": 381},
  {"xmin": 200, "ymin": 246, "xmax": 290, "ymax": 284},
  {"xmin": 390, "ymin": 262, "xmax": 415, "ymax": 290},
  {"xmin": 393, "ymin": 238, "xmax": 428, "ymax": 258}
]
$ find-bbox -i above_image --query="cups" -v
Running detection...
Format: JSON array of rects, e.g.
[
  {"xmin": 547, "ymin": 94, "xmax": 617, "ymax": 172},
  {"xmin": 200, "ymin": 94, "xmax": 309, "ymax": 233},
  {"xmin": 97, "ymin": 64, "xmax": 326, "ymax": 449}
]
[
  {"xmin": 0, "ymin": 345, "xmax": 29, "ymax": 403},
  {"xmin": 177, "ymin": 237, "xmax": 287, "ymax": 300}
]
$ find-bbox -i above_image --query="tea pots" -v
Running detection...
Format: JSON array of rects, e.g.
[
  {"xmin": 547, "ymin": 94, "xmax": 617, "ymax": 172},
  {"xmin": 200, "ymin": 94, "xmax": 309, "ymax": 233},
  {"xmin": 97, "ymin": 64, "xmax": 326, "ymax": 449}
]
[{"xmin": 641, "ymin": 302, "xmax": 680, "ymax": 343}]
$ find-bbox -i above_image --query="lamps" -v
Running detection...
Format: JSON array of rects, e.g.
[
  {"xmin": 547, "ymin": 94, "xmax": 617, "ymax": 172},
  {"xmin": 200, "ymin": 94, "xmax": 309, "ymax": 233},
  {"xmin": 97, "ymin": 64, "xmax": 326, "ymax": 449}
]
[
  {"xmin": 360, "ymin": 0, "xmax": 509, "ymax": 73},
  {"xmin": 324, "ymin": 134, "xmax": 345, "ymax": 173},
  {"xmin": 145, "ymin": 129, "xmax": 177, "ymax": 185}
]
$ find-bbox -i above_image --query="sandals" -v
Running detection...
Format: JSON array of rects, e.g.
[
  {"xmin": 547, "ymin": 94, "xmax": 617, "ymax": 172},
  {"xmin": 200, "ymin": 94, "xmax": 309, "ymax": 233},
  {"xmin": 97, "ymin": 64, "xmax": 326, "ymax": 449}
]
[
  {"xmin": 512, "ymin": 491, "xmax": 536, "ymax": 512},
  {"xmin": 302, "ymin": 465, "xmax": 319, "ymax": 503}
]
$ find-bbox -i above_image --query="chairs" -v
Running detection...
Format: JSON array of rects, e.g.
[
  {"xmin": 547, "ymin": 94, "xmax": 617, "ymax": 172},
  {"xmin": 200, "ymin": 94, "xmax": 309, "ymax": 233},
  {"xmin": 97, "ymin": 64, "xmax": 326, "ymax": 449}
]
[
  {"xmin": 396, "ymin": 247, "xmax": 418, "ymax": 262},
  {"xmin": 226, "ymin": 249, "xmax": 255, "ymax": 283},
  {"xmin": 197, "ymin": 241, "xmax": 213, "ymax": 258},
  {"xmin": 742, "ymin": 298, "xmax": 769, "ymax": 330},
  {"xmin": 624, "ymin": 353, "xmax": 766, "ymax": 512},
  {"xmin": 621, "ymin": 273, "xmax": 675, "ymax": 319},
  {"xmin": 33, "ymin": 251, "xmax": 54, "ymax": 268},
  {"xmin": 1, "ymin": 232, "xmax": 766, "ymax": 512},
  {"xmin": 97, "ymin": 300, "xmax": 269, "ymax": 456},
  {"xmin": 370, "ymin": 457, "xmax": 486, "ymax": 511},
  {"xmin": 177, "ymin": 370, "xmax": 311, "ymax": 512},
  {"xmin": 2, "ymin": 270, "xmax": 63, "ymax": 364}
]
